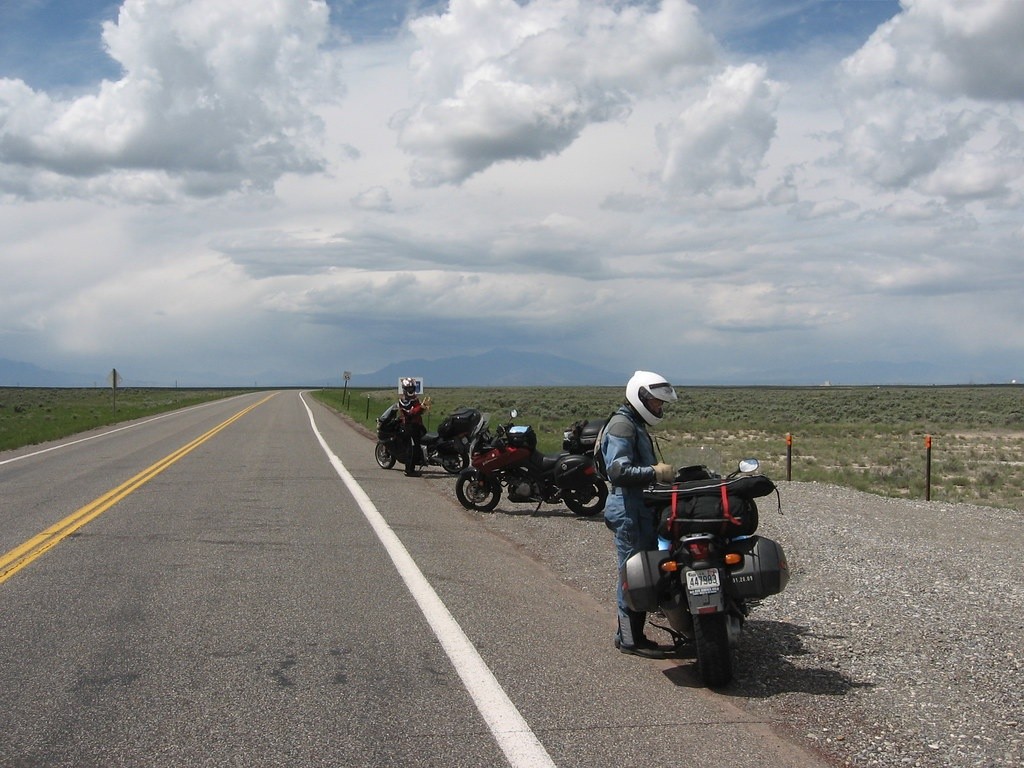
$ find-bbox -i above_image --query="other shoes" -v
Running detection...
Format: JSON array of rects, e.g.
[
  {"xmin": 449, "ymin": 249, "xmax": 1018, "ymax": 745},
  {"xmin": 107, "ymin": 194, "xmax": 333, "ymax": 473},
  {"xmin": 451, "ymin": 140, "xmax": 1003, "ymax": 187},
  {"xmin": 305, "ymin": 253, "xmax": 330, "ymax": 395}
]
[{"xmin": 403, "ymin": 470, "xmax": 421, "ymax": 477}]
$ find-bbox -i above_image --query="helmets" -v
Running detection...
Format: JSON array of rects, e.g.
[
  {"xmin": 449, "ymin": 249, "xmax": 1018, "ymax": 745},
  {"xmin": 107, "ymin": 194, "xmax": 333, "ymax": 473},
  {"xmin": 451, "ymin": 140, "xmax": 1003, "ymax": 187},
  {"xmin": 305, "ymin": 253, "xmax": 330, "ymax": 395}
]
[
  {"xmin": 626, "ymin": 371, "xmax": 678, "ymax": 426},
  {"xmin": 402, "ymin": 377, "xmax": 416, "ymax": 397}
]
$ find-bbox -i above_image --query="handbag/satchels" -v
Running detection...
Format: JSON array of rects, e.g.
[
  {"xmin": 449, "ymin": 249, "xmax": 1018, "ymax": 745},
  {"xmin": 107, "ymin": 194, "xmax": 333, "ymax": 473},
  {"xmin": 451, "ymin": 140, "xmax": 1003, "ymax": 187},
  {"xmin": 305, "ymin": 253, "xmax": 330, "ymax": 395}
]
[{"xmin": 643, "ymin": 476, "xmax": 783, "ymax": 540}]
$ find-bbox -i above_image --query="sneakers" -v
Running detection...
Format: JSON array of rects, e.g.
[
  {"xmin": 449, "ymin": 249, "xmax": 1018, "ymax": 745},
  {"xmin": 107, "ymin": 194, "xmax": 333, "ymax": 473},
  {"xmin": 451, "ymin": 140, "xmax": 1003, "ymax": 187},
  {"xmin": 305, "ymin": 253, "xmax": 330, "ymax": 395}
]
[{"xmin": 614, "ymin": 634, "xmax": 664, "ymax": 659}]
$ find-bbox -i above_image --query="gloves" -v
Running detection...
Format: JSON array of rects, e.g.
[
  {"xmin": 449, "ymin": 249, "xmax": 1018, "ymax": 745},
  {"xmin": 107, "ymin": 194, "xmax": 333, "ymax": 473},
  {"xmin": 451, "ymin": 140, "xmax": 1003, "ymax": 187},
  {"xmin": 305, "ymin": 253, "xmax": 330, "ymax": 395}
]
[
  {"xmin": 651, "ymin": 461, "xmax": 674, "ymax": 483},
  {"xmin": 421, "ymin": 397, "xmax": 432, "ymax": 410}
]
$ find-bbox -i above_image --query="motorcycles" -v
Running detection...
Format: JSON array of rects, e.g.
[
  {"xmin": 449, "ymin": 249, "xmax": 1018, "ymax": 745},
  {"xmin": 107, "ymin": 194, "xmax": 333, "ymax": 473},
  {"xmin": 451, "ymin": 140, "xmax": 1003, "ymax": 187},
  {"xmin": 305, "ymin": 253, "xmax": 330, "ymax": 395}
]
[
  {"xmin": 619, "ymin": 443, "xmax": 791, "ymax": 690},
  {"xmin": 374, "ymin": 401, "xmax": 482, "ymax": 475},
  {"xmin": 455, "ymin": 408, "xmax": 609, "ymax": 517}
]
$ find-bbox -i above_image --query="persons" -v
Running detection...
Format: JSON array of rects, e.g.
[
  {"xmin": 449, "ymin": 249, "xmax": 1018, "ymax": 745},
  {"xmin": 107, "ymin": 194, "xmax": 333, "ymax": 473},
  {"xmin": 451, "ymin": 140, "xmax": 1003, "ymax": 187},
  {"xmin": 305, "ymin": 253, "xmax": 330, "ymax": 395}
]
[
  {"xmin": 399, "ymin": 378, "xmax": 431, "ymax": 477},
  {"xmin": 600, "ymin": 371, "xmax": 677, "ymax": 659}
]
